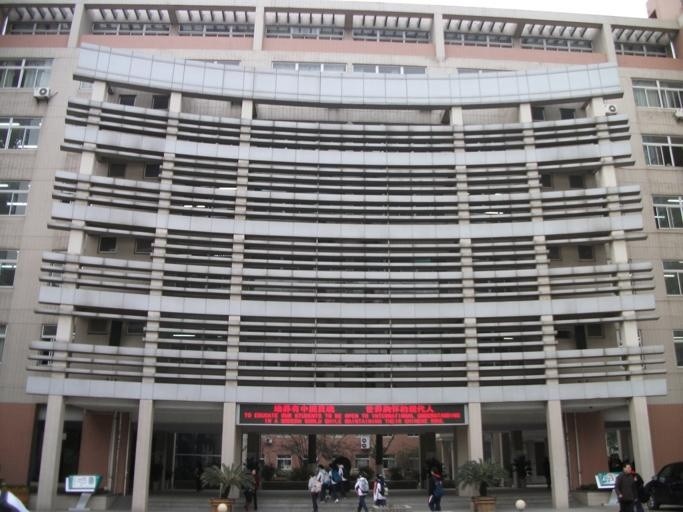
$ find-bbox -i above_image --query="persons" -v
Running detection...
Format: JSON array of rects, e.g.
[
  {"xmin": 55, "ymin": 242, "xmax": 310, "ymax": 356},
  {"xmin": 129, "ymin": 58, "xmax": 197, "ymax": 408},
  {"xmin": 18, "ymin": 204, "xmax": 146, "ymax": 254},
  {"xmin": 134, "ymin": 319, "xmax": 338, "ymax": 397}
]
[
  {"xmin": 614, "ymin": 462, "xmax": 638, "ymax": 511},
  {"xmin": 371, "ymin": 475, "xmax": 388, "ymax": 510},
  {"xmin": 426, "ymin": 466, "xmax": 441, "ymax": 512},
  {"xmin": 337, "ymin": 462, "xmax": 348, "ymax": 499},
  {"xmin": 373, "ymin": 473, "xmax": 380, "ymax": 500},
  {"xmin": 307, "ymin": 471, "xmax": 319, "ymax": 512},
  {"xmin": 240, "ymin": 467, "xmax": 258, "ymax": 511},
  {"xmin": 354, "ymin": 471, "xmax": 368, "ymax": 512},
  {"xmin": 315, "ymin": 464, "xmax": 327, "ymax": 503},
  {"xmin": 324, "ymin": 464, "xmax": 340, "ymax": 504}
]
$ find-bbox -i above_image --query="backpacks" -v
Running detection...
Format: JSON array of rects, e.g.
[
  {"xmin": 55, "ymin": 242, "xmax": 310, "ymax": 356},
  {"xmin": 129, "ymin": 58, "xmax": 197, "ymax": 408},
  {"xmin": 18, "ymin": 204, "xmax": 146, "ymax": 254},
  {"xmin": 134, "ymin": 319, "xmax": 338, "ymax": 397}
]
[
  {"xmin": 357, "ymin": 479, "xmax": 445, "ymax": 498},
  {"xmin": 310, "ymin": 466, "xmax": 349, "ymax": 493}
]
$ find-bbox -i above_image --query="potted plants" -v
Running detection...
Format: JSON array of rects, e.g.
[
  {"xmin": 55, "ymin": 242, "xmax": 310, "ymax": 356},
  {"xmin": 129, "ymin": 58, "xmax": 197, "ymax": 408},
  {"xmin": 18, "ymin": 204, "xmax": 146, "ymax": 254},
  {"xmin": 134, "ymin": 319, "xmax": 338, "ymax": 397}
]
[
  {"xmin": 200, "ymin": 462, "xmax": 257, "ymax": 512},
  {"xmin": 454, "ymin": 457, "xmax": 503, "ymax": 512}
]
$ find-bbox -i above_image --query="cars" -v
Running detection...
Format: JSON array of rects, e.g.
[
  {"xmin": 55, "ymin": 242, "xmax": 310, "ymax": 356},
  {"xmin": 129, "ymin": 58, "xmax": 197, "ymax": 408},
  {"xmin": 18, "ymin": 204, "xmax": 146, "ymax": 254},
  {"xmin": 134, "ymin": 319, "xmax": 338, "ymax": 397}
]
[{"xmin": 642, "ymin": 462, "xmax": 683, "ymax": 512}]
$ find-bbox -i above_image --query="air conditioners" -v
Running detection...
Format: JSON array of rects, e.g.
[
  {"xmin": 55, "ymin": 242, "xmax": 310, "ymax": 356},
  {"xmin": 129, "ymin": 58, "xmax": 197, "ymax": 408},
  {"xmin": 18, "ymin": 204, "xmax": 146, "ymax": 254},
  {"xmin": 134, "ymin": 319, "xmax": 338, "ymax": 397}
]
[
  {"xmin": 361, "ymin": 436, "xmax": 371, "ymax": 450},
  {"xmin": 605, "ymin": 103, "xmax": 617, "ymax": 114},
  {"xmin": 265, "ymin": 436, "xmax": 273, "ymax": 444},
  {"xmin": 34, "ymin": 87, "xmax": 51, "ymax": 100}
]
